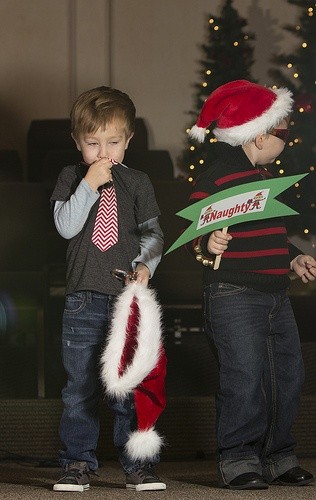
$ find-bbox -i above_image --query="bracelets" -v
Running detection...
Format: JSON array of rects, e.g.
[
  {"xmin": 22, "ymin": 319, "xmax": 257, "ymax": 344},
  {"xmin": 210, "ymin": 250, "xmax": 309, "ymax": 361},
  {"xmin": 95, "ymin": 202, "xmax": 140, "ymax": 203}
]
[{"xmin": 194, "ymin": 236, "xmax": 215, "ymax": 267}]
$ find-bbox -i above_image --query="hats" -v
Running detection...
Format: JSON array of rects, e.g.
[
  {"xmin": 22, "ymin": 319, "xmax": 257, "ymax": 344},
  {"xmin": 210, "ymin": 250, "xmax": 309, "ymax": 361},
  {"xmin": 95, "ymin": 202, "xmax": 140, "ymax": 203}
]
[
  {"xmin": 186, "ymin": 77, "xmax": 295, "ymax": 148},
  {"xmin": 95, "ymin": 280, "xmax": 170, "ymax": 467}
]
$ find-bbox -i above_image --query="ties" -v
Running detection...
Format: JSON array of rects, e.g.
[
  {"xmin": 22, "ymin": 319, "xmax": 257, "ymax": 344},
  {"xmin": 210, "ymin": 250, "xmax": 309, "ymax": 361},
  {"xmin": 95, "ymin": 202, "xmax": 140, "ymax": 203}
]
[{"xmin": 89, "ymin": 158, "xmax": 121, "ymax": 255}]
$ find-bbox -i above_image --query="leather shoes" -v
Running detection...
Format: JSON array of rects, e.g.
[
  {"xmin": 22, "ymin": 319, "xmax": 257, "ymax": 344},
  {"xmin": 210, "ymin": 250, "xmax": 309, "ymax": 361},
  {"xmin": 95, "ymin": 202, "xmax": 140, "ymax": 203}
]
[
  {"xmin": 275, "ymin": 464, "xmax": 314, "ymax": 487},
  {"xmin": 226, "ymin": 471, "xmax": 271, "ymax": 491}
]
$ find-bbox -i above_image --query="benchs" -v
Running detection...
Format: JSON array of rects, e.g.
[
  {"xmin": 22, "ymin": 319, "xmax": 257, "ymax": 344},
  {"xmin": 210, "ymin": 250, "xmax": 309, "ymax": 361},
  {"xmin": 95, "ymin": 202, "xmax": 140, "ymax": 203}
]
[{"xmin": 38, "ymin": 270, "xmax": 316, "ymax": 399}]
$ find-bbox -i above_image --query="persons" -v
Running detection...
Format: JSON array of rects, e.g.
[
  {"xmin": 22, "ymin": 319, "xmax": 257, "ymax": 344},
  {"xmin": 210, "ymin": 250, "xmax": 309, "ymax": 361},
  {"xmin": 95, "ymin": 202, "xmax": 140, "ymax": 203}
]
[
  {"xmin": 51, "ymin": 86, "xmax": 168, "ymax": 491},
  {"xmin": 180, "ymin": 80, "xmax": 316, "ymax": 490}
]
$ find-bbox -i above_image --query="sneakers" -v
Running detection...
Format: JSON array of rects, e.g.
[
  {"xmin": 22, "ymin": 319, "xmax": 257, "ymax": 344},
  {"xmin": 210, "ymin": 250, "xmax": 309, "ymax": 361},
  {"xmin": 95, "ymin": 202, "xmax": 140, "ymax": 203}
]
[
  {"xmin": 123, "ymin": 463, "xmax": 167, "ymax": 491},
  {"xmin": 51, "ymin": 460, "xmax": 91, "ymax": 493}
]
